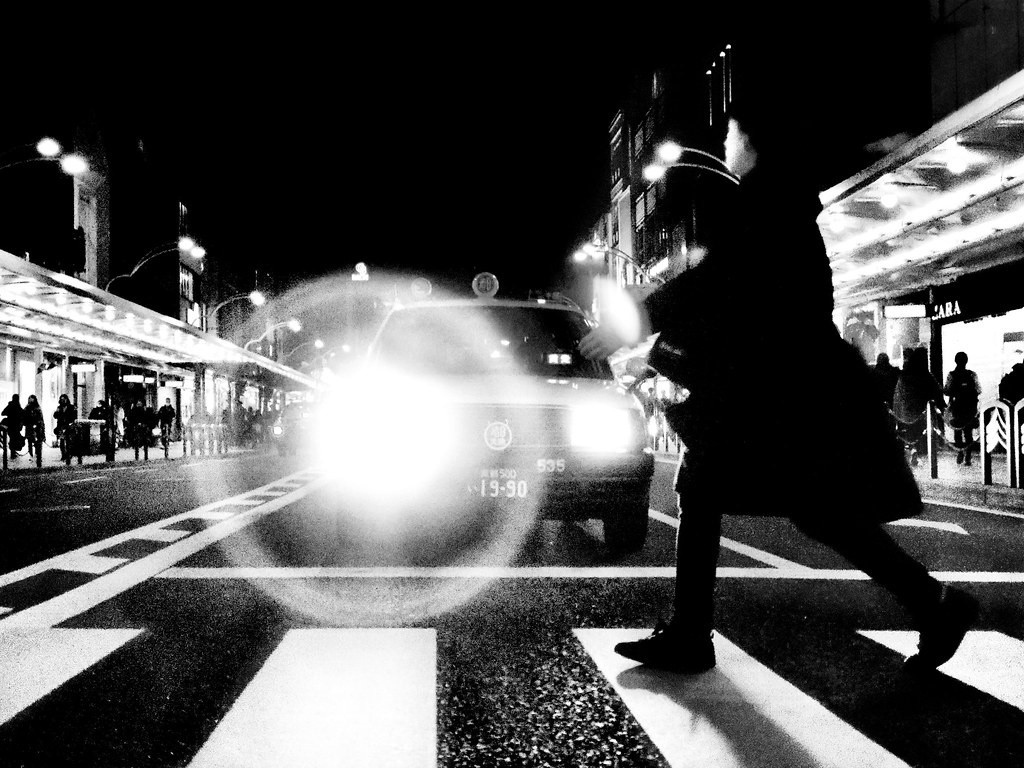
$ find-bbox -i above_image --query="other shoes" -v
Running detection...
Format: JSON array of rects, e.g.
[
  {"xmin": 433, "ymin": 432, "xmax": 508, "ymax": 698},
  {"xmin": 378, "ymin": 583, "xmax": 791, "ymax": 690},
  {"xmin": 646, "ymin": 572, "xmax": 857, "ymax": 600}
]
[
  {"xmin": 909, "ymin": 449, "xmax": 918, "ymax": 467},
  {"xmin": 905, "ymin": 581, "xmax": 981, "ymax": 669},
  {"xmin": 615, "ymin": 620, "xmax": 716, "ymax": 673},
  {"xmin": 956, "ymin": 451, "xmax": 965, "ymax": 464},
  {"xmin": 965, "ymin": 454, "xmax": 973, "ymax": 466}
]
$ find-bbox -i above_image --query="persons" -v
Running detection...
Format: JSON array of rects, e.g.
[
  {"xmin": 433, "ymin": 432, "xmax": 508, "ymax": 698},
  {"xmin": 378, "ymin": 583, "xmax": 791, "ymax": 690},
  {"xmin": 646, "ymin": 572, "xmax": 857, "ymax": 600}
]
[
  {"xmin": 575, "ymin": 92, "xmax": 979, "ymax": 676},
  {"xmin": 2, "ymin": 391, "xmax": 277, "ymax": 464},
  {"xmin": 645, "ymin": 346, "xmax": 1024, "ymax": 466}
]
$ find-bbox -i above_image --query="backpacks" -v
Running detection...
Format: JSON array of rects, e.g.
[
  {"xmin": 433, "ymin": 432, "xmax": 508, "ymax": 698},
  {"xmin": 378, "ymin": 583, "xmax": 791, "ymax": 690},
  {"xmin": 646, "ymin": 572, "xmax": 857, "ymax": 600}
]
[{"xmin": 949, "ymin": 370, "xmax": 978, "ymax": 419}]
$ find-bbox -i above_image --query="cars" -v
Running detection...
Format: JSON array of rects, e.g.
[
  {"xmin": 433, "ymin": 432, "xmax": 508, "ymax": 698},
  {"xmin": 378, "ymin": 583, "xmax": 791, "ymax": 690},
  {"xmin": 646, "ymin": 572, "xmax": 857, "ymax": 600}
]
[{"xmin": 357, "ymin": 297, "xmax": 658, "ymax": 550}]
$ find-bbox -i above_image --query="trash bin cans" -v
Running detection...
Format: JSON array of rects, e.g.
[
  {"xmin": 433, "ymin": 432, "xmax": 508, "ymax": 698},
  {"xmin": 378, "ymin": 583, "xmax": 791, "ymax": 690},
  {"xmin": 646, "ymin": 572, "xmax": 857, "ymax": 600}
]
[{"xmin": 74, "ymin": 425, "xmax": 91, "ymax": 455}]
